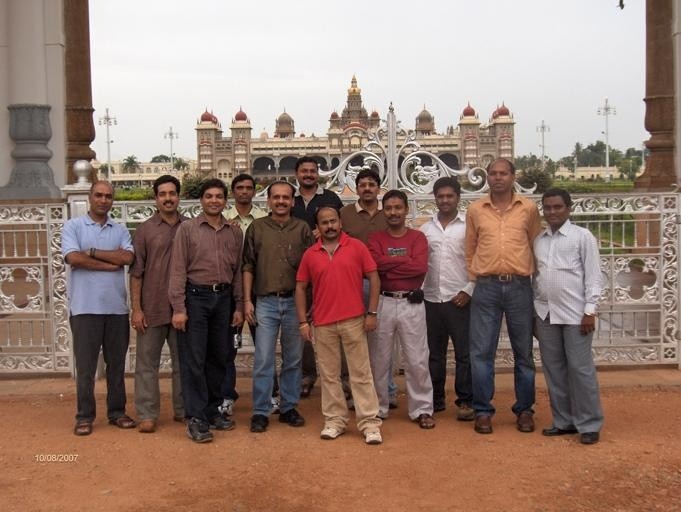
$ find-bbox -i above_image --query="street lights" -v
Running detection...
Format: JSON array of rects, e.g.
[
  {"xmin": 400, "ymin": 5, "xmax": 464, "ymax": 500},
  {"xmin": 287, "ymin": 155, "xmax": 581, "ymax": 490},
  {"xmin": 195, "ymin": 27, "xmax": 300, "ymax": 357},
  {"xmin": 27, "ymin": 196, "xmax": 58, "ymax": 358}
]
[
  {"xmin": 164, "ymin": 124, "xmax": 179, "ymax": 178},
  {"xmin": 597, "ymin": 96, "xmax": 617, "ymax": 183},
  {"xmin": 536, "ymin": 120, "xmax": 551, "ymax": 174},
  {"xmin": 98, "ymin": 106, "xmax": 118, "ymax": 185}
]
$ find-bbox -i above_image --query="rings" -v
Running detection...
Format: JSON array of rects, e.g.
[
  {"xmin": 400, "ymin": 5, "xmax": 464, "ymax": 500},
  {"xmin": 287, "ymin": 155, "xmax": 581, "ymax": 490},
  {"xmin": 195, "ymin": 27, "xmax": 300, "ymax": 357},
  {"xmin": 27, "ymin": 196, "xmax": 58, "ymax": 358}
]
[
  {"xmin": 458, "ymin": 303, "xmax": 461, "ymax": 305},
  {"xmin": 132, "ymin": 325, "xmax": 136, "ymax": 329},
  {"xmin": 456, "ymin": 300, "xmax": 458, "ymax": 303}
]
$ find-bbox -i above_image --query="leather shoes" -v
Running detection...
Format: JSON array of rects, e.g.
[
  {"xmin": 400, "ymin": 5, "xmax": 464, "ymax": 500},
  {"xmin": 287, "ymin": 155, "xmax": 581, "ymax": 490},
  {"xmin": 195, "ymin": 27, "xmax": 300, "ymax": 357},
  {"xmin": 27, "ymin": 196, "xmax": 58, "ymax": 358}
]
[
  {"xmin": 542, "ymin": 427, "xmax": 579, "ymax": 437},
  {"xmin": 580, "ymin": 431, "xmax": 600, "ymax": 444},
  {"xmin": 518, "ymin": 411, "xmax": 538, "ymax": 434},
  {"xmin": 473, "ymin": 414, "xmax": 495, "ymax": 434}
]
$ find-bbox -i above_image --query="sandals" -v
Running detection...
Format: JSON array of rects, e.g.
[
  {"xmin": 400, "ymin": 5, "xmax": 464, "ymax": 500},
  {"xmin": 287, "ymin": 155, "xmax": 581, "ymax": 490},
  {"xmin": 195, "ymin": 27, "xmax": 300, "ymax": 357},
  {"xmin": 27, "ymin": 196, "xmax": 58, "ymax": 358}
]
[
  {"xmin": 109, "ymin": 414, "xmax": 137, "ymax": 428},
  {"xmin": 74, "ymin": 419, "xmax": 95, "ymax": 436},
  {"xmin": 418, "ymin": 414, "xmax": 437, "ymax": 430},
  {"xmin": 455, "ymin": 401, "xmax": 476, "ymax": 421}
]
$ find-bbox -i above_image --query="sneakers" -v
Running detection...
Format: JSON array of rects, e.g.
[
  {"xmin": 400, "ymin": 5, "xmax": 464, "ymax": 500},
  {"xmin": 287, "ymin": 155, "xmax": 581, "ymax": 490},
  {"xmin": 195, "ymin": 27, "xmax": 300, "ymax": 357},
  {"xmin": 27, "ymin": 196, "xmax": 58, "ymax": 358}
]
[
  {"xmin": 174, "ymin": 395, "xmax": 308, "ymax": 442},
  {"xmin": 364, "ymin": 429, "xmax": 384, "ymax": 445},
  {"xmin": 318, "ymin": 425, "xmax": 345, "ymax": 440},
  {"xmin": 138, "ymin": 418, "xmax": 156, "ymax": 434}
]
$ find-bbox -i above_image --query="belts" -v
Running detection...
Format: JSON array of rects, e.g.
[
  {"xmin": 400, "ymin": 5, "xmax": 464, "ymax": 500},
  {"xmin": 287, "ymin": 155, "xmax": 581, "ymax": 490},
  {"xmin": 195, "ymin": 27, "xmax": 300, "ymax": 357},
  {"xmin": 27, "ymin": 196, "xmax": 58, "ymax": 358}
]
[
  {"xmin": 185, "ymin": 282, "xmax": 231, "ymax": 294},
  {"xmin": 379, "ymin": 290, "xmax": 413, "ymax": 299},
  {"xmin": 476, "ymin": 272, "xmax": 533, "ymax": 283},
  {"xmin": 266, "ymin": 288, "xmax": 296, "ymax": 299}
]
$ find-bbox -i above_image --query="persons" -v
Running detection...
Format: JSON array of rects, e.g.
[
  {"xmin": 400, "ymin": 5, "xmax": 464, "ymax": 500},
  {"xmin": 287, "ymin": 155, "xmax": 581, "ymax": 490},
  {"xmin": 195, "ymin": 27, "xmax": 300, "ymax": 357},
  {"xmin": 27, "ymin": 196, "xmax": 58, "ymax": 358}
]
[
  {"xmin": 418, "ymin": 177, "xmax": 476, "ymax": 422},
  {"xmin": 61, "ymin": 180, "xmax": 136, "ymax": 436},
  {"xmin": 129, "ymin": 174, "xmax": 193, "ymax": 432},
  {"xmin": 167, "ymin": 179, "xmax": 244, "ymax": 444},
  {"xmin": 529, "ymin": 188, "xmax": 605, "ymax": 444},
  {"xmin": 464, "ymin": 158, "xmax": 541, "ymax": 433}
]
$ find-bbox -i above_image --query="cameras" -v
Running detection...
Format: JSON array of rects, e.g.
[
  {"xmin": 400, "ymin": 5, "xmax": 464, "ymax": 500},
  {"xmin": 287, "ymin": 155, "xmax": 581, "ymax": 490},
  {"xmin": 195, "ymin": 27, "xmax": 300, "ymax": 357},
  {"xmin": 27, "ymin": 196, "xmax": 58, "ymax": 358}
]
[{"xmin": 234, "ymin": 334, "xmax": 242, "ymax": 348}]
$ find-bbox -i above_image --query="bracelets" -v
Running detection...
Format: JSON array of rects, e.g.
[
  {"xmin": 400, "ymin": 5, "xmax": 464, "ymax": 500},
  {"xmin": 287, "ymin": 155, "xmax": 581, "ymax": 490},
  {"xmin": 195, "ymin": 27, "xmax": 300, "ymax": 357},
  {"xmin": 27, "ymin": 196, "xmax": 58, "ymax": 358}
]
[{"xmin": 90, "ymin": 247, "xmax": 96, "ymax": 258}]
